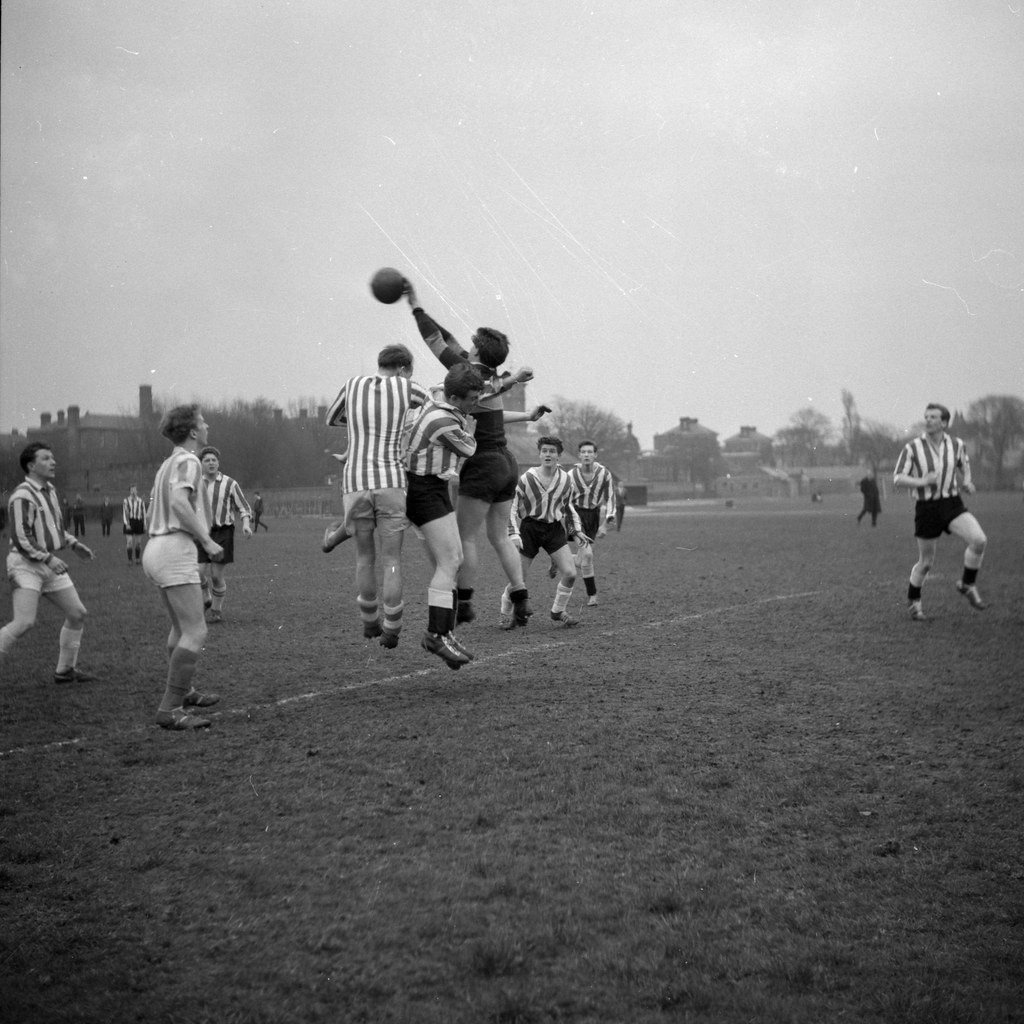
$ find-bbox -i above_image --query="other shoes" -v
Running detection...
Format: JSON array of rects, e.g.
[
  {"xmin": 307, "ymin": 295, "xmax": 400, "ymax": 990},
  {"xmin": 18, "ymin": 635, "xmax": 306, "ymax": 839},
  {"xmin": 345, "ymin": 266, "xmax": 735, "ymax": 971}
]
[
  {"xmin": 135, "ymin": 557, "xmax": 140, "ymax": 565},
  {"xmin": 128, "ymin": 558, "xmax": 133, "ymax": 566}
]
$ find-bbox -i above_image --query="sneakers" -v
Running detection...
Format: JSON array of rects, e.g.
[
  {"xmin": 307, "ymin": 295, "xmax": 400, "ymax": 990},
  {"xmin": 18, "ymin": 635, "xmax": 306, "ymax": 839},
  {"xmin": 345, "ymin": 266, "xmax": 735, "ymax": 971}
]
[
  {"xmin": 380, "ymin": 629, "xmax": 399, "ymax": 647},
  {"xmin": 363, "ymin": 620, "xmax": 382, "ymax": 638},
  {"xmin": 322, "ymin": 521, "xmax": 341, "ymax": 553},
  {"xmin": 905, "ymin": 598, "xmax": 927, "ymax": 622},
  {"xmin": 955, "ymin": 579, "xmax": 986, "ymax": 611},
  {"xmin": 499, "ymin": 613, "xmax": 511, "ymax": 629},
  {"xmin": 54, "ymin": 667, "xmax": 97, "ymax": 685},
  {"xmin": 206, "ymin": 608, "xmax": 222, "ymax": 623},
  {"xmin": 455, "ymin": 601, "xmax": 475, "ymax": 625},
  {"xmin": 549, "ymin": 557, "xmax": 559, "ymax": 579},
  {"xmin": 512, "ymin": 598, "xmax": 533, "ymax": 628},
  {"xmin": 183, "ymin": 685, "xmax": 220, "ymax": 709},
  {"xmin": 422, "ymin": 630, "xmax": 473, "ymax": 662},
  {"xmin": 587, "ymin": 594, "xmax": 599, "ymax": 606},
  {"xmin": 155, "ymin": 706, "xmax": 211, "ymax": 729},
  {"xmin": 204, "ymin": 599, "xmax": 213, "ymax": 611},
  {"xmin": 550, "ymin": 609, "xmax": 580, "ymax": 625}
]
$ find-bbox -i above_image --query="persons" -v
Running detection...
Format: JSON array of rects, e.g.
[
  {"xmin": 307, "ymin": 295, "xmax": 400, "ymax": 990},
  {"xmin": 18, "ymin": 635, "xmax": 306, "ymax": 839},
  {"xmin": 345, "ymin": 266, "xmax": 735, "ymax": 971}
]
[
  {"xmin": 547, "ymin": 441, "xmax": 617, "ymax": 606},
  {"xmin": 140, "ymin": 404, "xmax": 220, "ymax": 730},
  {"xmin": 321, "ymin": 276, "xmax": 553, "ymax": 664},
  {"xmin": 0, "ymin": 442, "xmax": 101, "ymax": 684},
  {"xmin": 62, "ymin": 447, "xmax": 268, "ymax": 624},
  {"xmin": 615, "ymin": 481, "xmax": 628, "ymax": 532},
  {"xmin": 893, "ymin": 403, "xmax": 988, "ymax": 621},
  {"xmin": 855, "ymin": 470, "xmax": 881, "ymax": 527},
  {"xmin": 500, "ymin": 436, "xmax": 595, "ymax": 630}
]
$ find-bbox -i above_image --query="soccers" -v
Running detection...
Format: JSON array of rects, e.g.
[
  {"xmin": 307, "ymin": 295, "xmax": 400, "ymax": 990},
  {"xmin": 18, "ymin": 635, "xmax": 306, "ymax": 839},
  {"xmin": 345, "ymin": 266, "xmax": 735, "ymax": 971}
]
[{"xmin": 373, "ymin": 267, "xmax": 404, "ymax": 305}]
